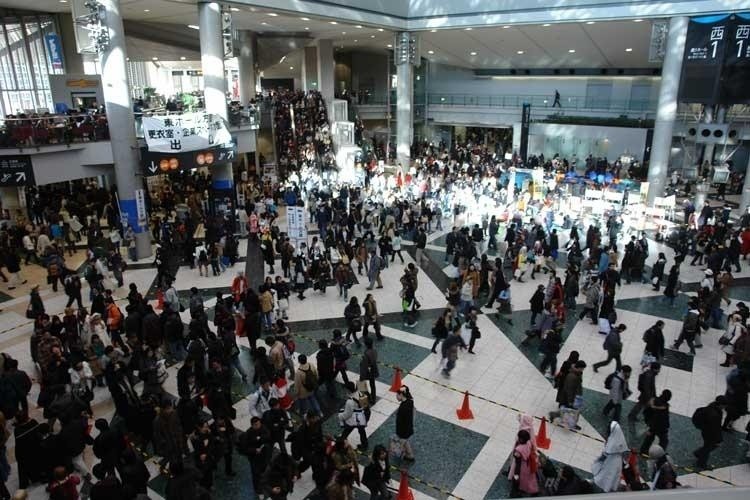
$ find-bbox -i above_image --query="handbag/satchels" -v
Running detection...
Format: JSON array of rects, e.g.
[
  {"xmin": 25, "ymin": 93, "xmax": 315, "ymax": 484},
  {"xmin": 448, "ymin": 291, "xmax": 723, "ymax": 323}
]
[
  {"xmin": 231, "ymin": 342, "xmax": 240, "ymax": 358},
  {"xmin": 338, "ymin": 409, "xmax": 367, "ymax": 428},
  {"xmin": 432, "ymin": 326, "xmax": 448, "ymax": 339},
  {"xmin": 27, "ymin": 310, "xmax": 42, "ymax": 318},
  {"xmin": 360, "ymin": 364, "xmax": 378, "ymax": 378},
  {"xmin": 558, "ymin": 404, "xmax": 579, "ymax": 429},
  {"xmin": 530, "ymin": 451, "xmax": 538, "ymax": 473},
  {"xmin": 139, "ymin": 371, "xmax": 148, "ymax": 381}
]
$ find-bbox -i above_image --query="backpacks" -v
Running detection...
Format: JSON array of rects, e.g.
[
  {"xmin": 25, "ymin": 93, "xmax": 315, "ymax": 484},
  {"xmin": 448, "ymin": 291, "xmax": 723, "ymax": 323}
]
[
  {"xmin": 199, "ymin": 249, "xmax": 207, "ymax": 262},
  {"xmin": 638, "ymin": 372, "xmax": 647, "ymax": 392},
  {"xmin": 50, "ymin": 264, "xmax": 58, "ymax": 276},
  {"xmin": 351, "ymin": 318, "xmax": 361, "ymax": 332},
  {"xmin": 349, "ymin": 393, "xmax": 371, "ymax": 422},
  {"xmin": 605, "ymin": 371, "xmax": 625, "ymax": 389},
  {"xmin": 335, "ymin": 341, "xmax": 349, "ymax": 361},
  {"xmin": 298, "ymin": 363, "xmax": 318, "ymax": 392},
  {"xmin": 286, "ymin": 335, "xmax": 295, "ymax": 355}
]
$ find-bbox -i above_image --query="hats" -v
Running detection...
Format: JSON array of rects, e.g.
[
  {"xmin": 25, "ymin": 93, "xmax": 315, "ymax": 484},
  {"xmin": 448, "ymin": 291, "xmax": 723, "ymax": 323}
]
[
  {"xmin": 704, "ymin": 269, "xmax": 713, "ymax": 275},
  {"xmin": 31, "ymin": 284, "xmax": 39, "ymax": 290},
  {"xmin": 398, "ymin": 385, "xmax": 413, "ymax": 399},
  {"xmin": 649, "ymin": 445, "xmax": 666, "ymax": 458}
]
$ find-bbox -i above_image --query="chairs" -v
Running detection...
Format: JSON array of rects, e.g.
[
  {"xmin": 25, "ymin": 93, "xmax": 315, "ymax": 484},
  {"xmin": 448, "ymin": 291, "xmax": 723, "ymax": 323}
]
[{"xmin": 0, "ymin": 116, "xmax": 108, "ymax": 145}]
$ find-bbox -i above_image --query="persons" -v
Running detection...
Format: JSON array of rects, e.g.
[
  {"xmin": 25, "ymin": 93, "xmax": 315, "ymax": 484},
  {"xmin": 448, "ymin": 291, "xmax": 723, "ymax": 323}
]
[
  {"xmin": 1, "ymin": 102, "xmax": 110, "ymax": 150},
  {"xmin": 551, "ymin": 89, "xmax": 562, "ymax": 108},
  {"xmin": 504, "ymin": 300, "xmax": 750, "ymax": 489},
  {"xmin": 268, "ymin": 88, "xmax": 332, "ymax": 166},
  {"xmin": 411, "ymin": 133, "xmax": 645, "ymax": 218},
  {"xmin": 2, "ymin": 280, "xmax": 415, "ymax": 500},
  {"xmin": 228, "ymin": 92, "xmax": 264, "ymax": 128},
  {"xmin": 242, "ymin": 152, "xmax": 432, "ymax": 303},
  {"xmin": 400, "ymin": 214, "xmax": 622, "ymax": 379},
  {"xmin": 1, "ymin": 177, "xmax": 128, "ymax": 308},
  {"xmin": 147, "ymin": 170, "xmax": 240, "ymax": 278},
  {"xmin": 619, "ymin": 200, "xmax": 750, "ymax": 308},
  {"xmin": 669, "ymin": 169, "xmax": 746, "ymax": 200},
  {"xmin": 335, "ymin": 89, "xmax": 370, "ymax": 105},
  {"xmin": 133, "ymin": 95, "xmax": 203, "ymax": 119}
]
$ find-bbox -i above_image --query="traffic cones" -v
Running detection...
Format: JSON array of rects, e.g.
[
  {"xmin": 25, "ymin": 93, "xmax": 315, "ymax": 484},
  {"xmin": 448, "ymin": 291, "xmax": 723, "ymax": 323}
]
[
  {"xmin": 388, "ymin": 368, "xmax": 403, "ymax": 393},
  {"xmin": 621, "ymin": 448, "xmax": 641, "ymax": 481},
  {"xmin": 153, "ymin": 288, "xmax": 164, "ymax": 310},
  {"xmin": 536, "ymin": 416, "xmax": 552, "ymax": 451},
  {"xmin": 395, "ymin": 469, "xmax": 413, "ymax": 500},
  {"xmin": 455, "ymin": 389, "xmax": 474, "ymax": 420}
]
